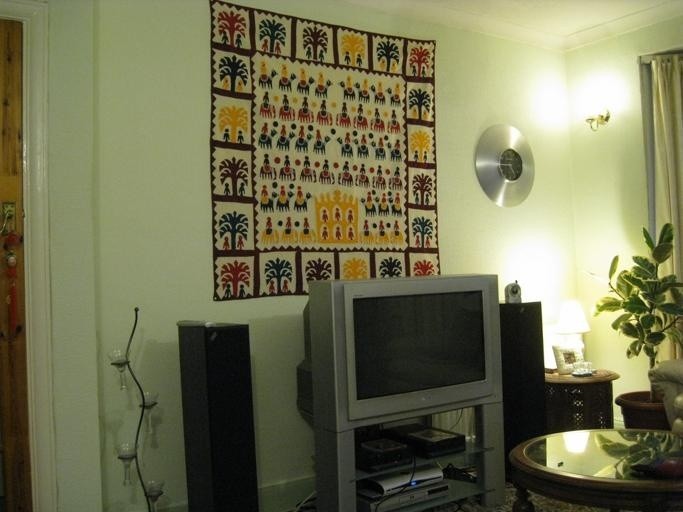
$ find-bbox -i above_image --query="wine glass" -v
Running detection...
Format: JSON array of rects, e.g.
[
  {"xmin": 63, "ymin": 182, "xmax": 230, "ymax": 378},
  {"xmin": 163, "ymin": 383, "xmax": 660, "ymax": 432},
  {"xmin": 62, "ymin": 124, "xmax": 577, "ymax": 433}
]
[
  {"xmin": 142, "ymin": 475, "xmax": 165, "ymax": 511},
  {"xmin": 137, "ymin": 390, "xmax": 161, "ymax": 434},
  {"xmin": 106, "ymin": 343, "xmax": 135, "ymax": 394},
  {"xmin": 115, "ymin": 440, "xmax": 139, "ymax": 488}
]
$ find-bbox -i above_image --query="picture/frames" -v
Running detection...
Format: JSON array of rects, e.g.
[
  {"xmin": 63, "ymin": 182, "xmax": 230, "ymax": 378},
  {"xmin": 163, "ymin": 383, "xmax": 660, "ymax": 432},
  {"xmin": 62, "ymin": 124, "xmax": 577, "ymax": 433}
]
[{"xmin": 553, "ymin": 345, "xmax": 583, "ymax": 375}]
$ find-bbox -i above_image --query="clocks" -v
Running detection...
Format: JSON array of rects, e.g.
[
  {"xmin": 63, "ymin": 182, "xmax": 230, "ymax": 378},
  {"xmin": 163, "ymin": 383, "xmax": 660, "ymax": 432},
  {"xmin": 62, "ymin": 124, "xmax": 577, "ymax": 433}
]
[{"xmin": 474, "ymin": 124, "xmax": 535, "ymax": 210}]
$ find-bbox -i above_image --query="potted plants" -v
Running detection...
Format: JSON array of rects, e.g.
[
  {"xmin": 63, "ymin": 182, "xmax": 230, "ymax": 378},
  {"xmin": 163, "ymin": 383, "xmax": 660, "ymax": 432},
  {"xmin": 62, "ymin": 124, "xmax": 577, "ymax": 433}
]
[{"xmin": 591, "ymin": 223, "xmax": 682, "ymax": 428}]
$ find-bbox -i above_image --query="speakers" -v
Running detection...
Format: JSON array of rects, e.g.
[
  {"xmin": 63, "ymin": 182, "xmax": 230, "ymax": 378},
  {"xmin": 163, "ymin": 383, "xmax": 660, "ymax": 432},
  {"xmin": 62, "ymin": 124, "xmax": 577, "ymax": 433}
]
[
  {"xmin": 177, "ymin": 323, "xmax": 259, "ymax": 511},
  {"xmin": 500, "ymin": 302, "xmax": 546, "ymax": 482}
]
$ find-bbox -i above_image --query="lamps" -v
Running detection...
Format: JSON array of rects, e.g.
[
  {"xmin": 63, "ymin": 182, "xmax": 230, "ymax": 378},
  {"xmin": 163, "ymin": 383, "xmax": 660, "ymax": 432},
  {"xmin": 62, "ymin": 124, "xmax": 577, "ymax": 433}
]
[{"xmin": 555, "ymin": 299, "xmax": 591, "ymax": 361}]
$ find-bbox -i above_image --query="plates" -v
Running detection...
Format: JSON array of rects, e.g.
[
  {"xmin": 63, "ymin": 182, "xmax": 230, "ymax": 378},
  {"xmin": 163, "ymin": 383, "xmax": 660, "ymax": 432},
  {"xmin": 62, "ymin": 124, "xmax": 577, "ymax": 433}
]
[{"xmin": 571, "ymin": 372, "xmax": 593, "ymax": 377}]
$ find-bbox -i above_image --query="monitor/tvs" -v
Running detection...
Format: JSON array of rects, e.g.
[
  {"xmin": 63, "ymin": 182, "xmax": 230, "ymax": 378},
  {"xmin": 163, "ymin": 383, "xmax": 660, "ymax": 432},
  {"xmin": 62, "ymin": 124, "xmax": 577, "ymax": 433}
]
[{"xmin": 297, "ymin": 273, "xmax": 503, "ymax": 433}]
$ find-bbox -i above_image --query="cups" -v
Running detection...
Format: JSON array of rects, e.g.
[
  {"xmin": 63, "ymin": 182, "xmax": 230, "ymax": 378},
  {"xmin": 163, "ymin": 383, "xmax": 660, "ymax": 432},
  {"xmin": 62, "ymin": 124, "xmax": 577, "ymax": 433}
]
[{"xmin": 572, "ymin": 361, "xmax": 592, "ymax": 373}]
